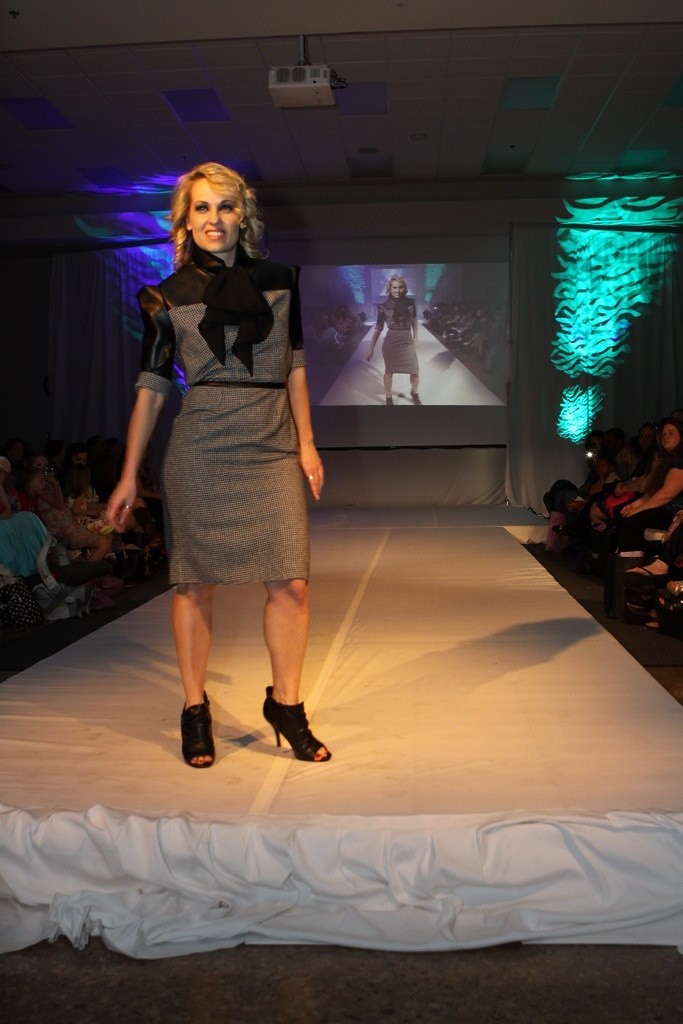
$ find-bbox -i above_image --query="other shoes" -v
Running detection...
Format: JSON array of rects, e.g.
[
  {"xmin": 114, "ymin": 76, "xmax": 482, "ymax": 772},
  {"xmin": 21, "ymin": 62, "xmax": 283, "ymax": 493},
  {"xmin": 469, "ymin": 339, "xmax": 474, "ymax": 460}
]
[
  {"xmin": 85, "ymin": 591, "xmax": 114, "ymax": 608},
  {"xmin": 627, "ymin": 602, "xmax": 654, "ymax": 619}
]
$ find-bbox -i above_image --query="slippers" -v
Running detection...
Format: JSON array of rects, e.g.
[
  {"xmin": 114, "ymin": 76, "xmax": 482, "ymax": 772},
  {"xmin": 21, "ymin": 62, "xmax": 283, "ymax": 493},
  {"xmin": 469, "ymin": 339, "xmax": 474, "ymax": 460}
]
[{"xmin": 624, "ymin": 567, "xmax": 671, "ymax": 585}]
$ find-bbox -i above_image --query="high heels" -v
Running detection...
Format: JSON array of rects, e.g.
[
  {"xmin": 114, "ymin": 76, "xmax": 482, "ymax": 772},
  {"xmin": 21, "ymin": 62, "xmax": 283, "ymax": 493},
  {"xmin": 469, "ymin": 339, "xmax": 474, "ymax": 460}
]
[
  {"xmin": 263, "ymin": 687, "xmax": 331, "ymax": 761},
  {"xmin": 181, "ymin": 692, "xmax": 214, "ymax": 768},
  {"xmin": 387, "ymin": 394, "xmax": 393, "ymax": 405},
  {"xmin": 410, "ymin": 391, "xmax": 421, "ymax": 406}
]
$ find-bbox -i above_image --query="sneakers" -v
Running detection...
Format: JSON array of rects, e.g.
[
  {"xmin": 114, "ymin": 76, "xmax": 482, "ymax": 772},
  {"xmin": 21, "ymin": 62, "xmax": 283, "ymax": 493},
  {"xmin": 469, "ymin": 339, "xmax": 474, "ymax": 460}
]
[{"xmin": 96, "ymin": 576, "xmax": 124, "ymax": 589}]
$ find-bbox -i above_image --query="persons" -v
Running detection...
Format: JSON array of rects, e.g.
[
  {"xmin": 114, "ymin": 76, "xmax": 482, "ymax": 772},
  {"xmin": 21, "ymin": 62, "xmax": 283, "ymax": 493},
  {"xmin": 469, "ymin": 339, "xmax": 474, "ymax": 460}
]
[
  {"xmin": 104, "ymin": 162, "xmax": 334, "ymax": 772},
  {"xmin": 365, "ymin": 275, "xmax": 422, "ymax": 409},
  {"xmin": 544, "ymin": 413, "xmax": 683, "ymax": 642},
  {"xmin": 313, "ymin": 303, "xmax": 364, "ymax": 362},
  {"xmin": 426, "ymin": 298, "xmax": 510, "ymax": 381},
  {"xmin": 0, "ymin": 434, "xmax": 174, "ymax": 633}
]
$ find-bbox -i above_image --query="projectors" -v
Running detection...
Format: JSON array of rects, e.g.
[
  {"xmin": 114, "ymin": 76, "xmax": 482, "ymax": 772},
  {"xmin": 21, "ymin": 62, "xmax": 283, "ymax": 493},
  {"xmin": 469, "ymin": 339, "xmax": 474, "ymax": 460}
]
[{"xmin": 267, "ymin": 66, "xmax": 336, "ymax": 108}]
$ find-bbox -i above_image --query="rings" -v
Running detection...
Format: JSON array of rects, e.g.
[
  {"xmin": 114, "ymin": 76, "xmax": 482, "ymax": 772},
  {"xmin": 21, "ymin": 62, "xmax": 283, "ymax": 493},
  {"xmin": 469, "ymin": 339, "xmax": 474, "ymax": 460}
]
[
  {"xmin": 123, "ymin": 504, "xmax": 132, "ymax": 510},
  {"xmin": 307, "ymin": 476, "xmax": 314, "ymax": 481}
]
